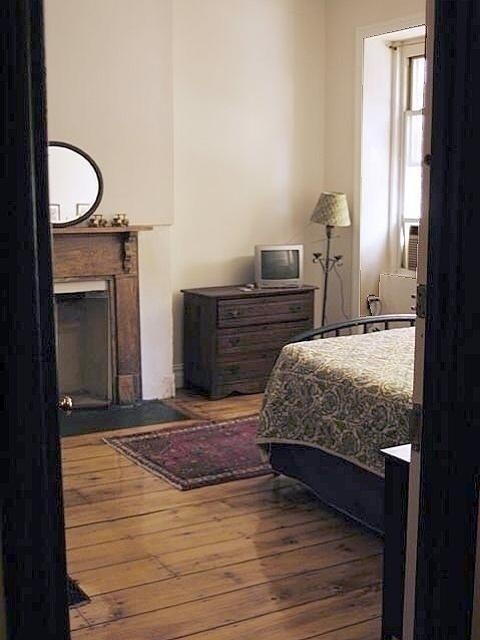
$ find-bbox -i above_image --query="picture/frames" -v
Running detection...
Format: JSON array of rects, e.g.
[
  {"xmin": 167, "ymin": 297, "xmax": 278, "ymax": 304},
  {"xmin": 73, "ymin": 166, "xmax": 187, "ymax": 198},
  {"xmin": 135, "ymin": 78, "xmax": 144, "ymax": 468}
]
[
  {"xmin": 49, "ymin": 203, "xmax": 62, "ymax": 223},
  {"xmin": 74, "ymin": 202, "xmax": 92, "ymax": 217}
]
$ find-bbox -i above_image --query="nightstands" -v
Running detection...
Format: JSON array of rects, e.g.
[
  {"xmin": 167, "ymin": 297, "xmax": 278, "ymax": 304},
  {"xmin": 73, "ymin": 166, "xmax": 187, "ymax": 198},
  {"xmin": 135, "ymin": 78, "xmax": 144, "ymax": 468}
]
[{"xmin": 374, "ymin": 440, "xmax": 412, "ymax": 640}]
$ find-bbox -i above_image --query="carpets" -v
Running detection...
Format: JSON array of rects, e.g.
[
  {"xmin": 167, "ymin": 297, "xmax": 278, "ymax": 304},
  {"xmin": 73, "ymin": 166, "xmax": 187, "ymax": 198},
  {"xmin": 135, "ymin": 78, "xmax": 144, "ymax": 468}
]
[
  {"xmin": 59, "ymin": 393, "xmax": 193, "ymax": 439},
  {"xmin": 99, "ymin": 414, "xmax": 277, "ymax": 493}
]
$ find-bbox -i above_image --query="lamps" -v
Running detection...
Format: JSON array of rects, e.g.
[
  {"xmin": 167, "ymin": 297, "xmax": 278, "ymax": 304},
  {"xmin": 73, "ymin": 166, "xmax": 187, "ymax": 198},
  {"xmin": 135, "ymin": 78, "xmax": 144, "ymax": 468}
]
[{"xmin": 304, "ymin": 190, "xmax": 353, "ymax": 329}]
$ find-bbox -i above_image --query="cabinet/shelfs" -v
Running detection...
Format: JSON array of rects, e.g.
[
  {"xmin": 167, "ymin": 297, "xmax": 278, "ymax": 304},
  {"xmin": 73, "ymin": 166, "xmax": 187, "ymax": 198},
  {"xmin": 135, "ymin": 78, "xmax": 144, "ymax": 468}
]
[{"xmin": 178, "ymin": 282, "xmax": 320, "ymax": 404}]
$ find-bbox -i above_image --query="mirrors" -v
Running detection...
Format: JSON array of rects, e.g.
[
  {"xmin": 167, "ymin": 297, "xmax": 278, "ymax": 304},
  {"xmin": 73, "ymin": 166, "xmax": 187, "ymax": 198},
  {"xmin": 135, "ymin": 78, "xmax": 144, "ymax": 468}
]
[{"xmin": 46, "ymin": 139, "xmax": 107, "ymax": 229}]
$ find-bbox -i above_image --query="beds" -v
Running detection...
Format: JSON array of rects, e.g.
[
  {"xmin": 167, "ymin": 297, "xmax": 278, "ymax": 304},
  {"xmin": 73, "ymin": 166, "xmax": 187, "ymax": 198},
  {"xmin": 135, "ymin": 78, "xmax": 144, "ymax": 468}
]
[{"xmin": 253, "ymin": 313, "xmax": 415, "ymax": 541}]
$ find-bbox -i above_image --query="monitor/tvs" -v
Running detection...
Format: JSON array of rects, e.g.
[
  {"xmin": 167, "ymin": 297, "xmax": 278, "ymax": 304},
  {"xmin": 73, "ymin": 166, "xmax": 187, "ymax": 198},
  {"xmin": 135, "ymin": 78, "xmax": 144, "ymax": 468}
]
[{"xmin": 255, "ymin": 244, "xmax": 303, "ymax": 289}]
